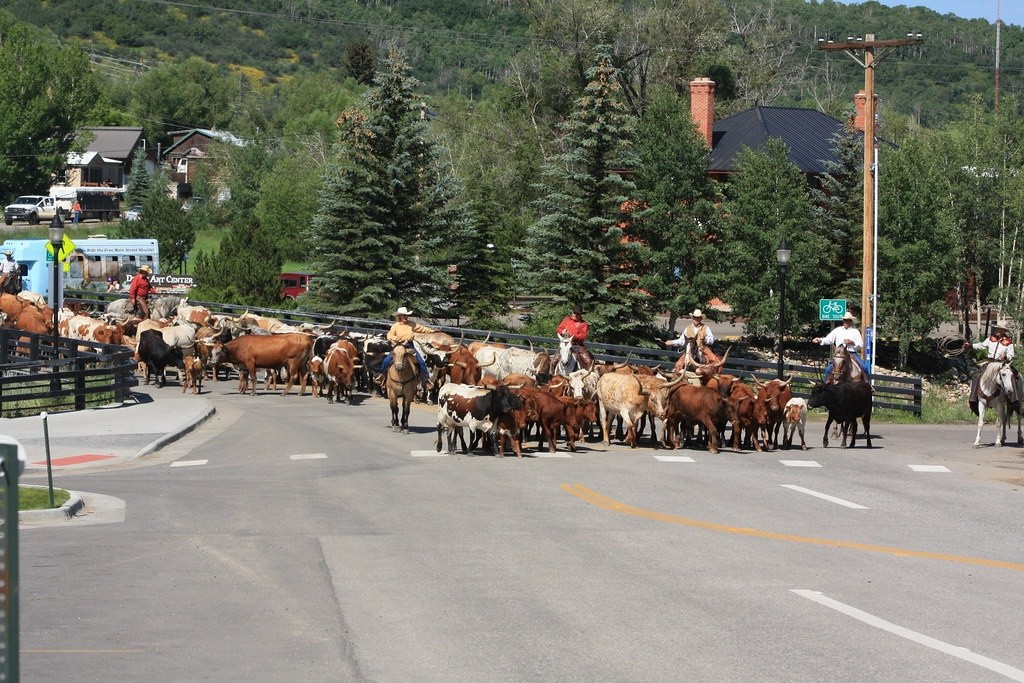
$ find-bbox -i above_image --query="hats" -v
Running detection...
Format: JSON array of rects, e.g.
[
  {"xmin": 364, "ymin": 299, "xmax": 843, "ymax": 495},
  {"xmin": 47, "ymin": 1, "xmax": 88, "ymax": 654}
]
[
  {"xmin": 689, "ymin": 309, "xmax": 707, "ymax": 321},
  {"xmin": 108, "ymin": 277, "xmax": 112, "ymax": 282},
  {"xmin": 392, "ymin": 307, "xmax": 413, "ymax": 316},
  {"xmin": 991, "ymin": 321, "xmax": 1010, "ymax": 332},
  {"xmin": 842, "ymin": 312, "xmax": 858, "ymax": 322},
  {"xmin": 570, "ymin": 304, "xmax": 587, "ymax": 315},
  {"xmin": 138, "ymin": 264, "xmax": 152, "ymax": 274},
  {"xmin": 6, "ymin": 251, "xmax": 13, "ymax": 255}
]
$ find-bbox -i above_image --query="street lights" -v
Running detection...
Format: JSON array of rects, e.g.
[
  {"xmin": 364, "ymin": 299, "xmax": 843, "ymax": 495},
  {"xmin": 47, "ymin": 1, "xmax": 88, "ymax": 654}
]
[
  {"xmin": 775, "ymin": 237, "xmax": 792, "ymax": 380},
  {"xmin": 48, "ymin": 211, "xmax": 69, "ymax": 397}
]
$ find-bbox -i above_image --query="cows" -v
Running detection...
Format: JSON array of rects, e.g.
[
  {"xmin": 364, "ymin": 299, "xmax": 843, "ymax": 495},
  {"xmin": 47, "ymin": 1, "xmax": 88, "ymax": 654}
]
[
  {"xmin": 0, "ymin": 285, "xmax": 808, "ymax": 460},
  {"xmin": 386, "ymin": 347, "xmax": 420, "ymax": 433},
  {"xmin": 808, "ymin": 380, "xmax": 872, "ymax": 450}
]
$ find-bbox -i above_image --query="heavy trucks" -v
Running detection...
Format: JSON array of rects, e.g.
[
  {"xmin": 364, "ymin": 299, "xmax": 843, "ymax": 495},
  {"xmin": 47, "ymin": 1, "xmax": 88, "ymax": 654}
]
[{"xmin": 2, "ymin": 185, "xmax": 122, "ymax": 226}]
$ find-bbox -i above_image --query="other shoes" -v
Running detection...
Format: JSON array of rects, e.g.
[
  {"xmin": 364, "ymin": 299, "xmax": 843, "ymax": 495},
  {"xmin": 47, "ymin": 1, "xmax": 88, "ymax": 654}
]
[
  {"xmin": 424, "ymin": 382, "xmax": 433, "ymax": 390},
  {"xmin": 375, "ymin": 373, "xmax": 383, "ymax": 382},
  {"xmin": 871, "ymin": 385, "xmax": 875, "ymax": 395}
]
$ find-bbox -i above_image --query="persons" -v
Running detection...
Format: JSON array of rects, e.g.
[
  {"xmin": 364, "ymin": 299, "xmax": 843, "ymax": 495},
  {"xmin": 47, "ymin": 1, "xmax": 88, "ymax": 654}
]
[
  {"xmin": 129, "ymin": 265, "xmax": 161, "ymax": 319},
  {"xmin": 552, "ymin": 307, "xmax": 592, "ymax": 370},
  {"xmin": 664, "ymin": 309, "xmax": 721, "ymax": 370},
  {"xmin": 965, "ymin": 325, "xmax": 1018, "ymax": 403},
  {"xmin": 106, "ymin": 278, "xmax": 122, "ymax": 293},
  {"xmin": 0, "ymin": 251, "xmax": 19, "ymax": 273},
  {"xmin": 375, "ymin": 307, "xmax": 440, "ymax": 390},
  {"xmin": 70, "ymin": 201, "xmax": 82, "ymax": 224},
  {"xmin": 813, "ymin": 312, "xmax": 876, "ymax": 395}
]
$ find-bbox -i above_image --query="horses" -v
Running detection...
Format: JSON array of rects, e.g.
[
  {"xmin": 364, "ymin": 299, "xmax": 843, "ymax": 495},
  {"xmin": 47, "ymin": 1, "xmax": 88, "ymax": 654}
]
[
  {"xmin": 680, "ymin": 333, "xmax": 704, "ymax": 372},
  {"xmin": 973, "ymin": 361, "xmax": 1024, "ymax": 449},
  {"xmin": 830, "ymin": 343, "xmax": 869, "ymax": 441},
  {"xmin": 554, "ymin": 332, "xmax": 582, "ymax": 377}
]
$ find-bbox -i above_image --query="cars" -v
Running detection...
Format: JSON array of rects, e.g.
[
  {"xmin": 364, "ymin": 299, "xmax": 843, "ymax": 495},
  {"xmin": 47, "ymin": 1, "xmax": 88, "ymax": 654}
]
[{"xmin": 122, "ymin": 204, "xmax": 143, "ymax": 223}]
